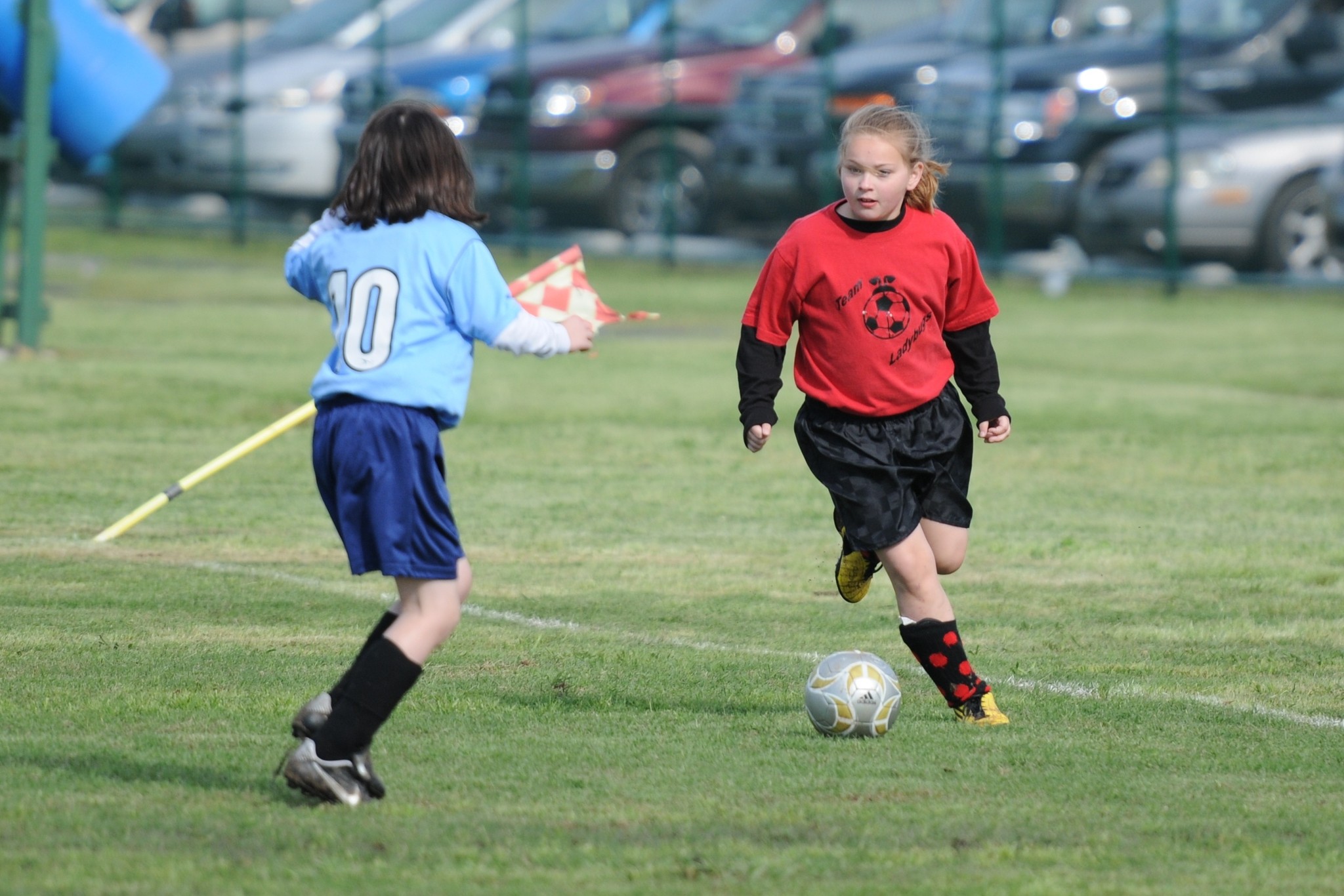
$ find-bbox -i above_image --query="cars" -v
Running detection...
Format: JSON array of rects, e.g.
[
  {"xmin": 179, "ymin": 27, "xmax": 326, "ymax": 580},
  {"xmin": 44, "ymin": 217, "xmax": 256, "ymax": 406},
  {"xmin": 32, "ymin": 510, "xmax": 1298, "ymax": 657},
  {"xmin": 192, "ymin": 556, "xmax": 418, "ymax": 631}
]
[{"xmin": 94, "ymin": 0, "xmax": 1344, "ymax": 286}]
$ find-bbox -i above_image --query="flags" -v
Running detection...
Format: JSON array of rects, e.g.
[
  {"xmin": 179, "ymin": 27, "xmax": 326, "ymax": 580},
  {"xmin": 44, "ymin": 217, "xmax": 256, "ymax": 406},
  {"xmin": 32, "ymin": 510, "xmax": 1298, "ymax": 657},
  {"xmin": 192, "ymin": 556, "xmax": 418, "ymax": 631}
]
[{"xmin": 514, "ymin": 259, "xmax": 660, "ymax": 358}]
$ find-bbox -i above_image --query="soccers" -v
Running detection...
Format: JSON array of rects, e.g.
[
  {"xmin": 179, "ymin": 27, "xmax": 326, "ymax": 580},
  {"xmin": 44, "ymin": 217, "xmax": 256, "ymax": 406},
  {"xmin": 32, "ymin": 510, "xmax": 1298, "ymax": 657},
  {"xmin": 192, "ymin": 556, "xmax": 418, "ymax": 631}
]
[{"xmin": 804, "ymin": 651, "xmax": 902, "ymax": 738}]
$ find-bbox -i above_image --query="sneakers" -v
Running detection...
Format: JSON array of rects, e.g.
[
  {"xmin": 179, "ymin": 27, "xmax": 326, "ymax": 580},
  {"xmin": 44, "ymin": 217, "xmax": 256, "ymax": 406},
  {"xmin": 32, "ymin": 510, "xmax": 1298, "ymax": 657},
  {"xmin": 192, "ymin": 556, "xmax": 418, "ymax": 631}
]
[
  {"xmin": 832, "ymin": 505, "xmax": 882, "ymax": 604},
  {"xmin": 952, "ymin": 690, "xmax": 1012, "ymax": 728},
  {"xmin": 282, "ymin": 736, "xmax": 373, "ymax": 812},
  {"xmin": 289, "ymin": 690, "xmax": 386, "ymax": 801}
]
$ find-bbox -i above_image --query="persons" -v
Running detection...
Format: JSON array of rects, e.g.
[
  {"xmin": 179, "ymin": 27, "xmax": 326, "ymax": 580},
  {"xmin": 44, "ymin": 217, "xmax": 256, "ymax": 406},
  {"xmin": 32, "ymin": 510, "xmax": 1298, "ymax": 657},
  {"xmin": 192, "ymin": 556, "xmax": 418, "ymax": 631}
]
[
  {"xmin": 734, "ymin": 106, "xmax": 1015, "ymax": 724},
  {"xmin": 279, "ymin": 103, "xmax": 590, "ymax": 807}
]
